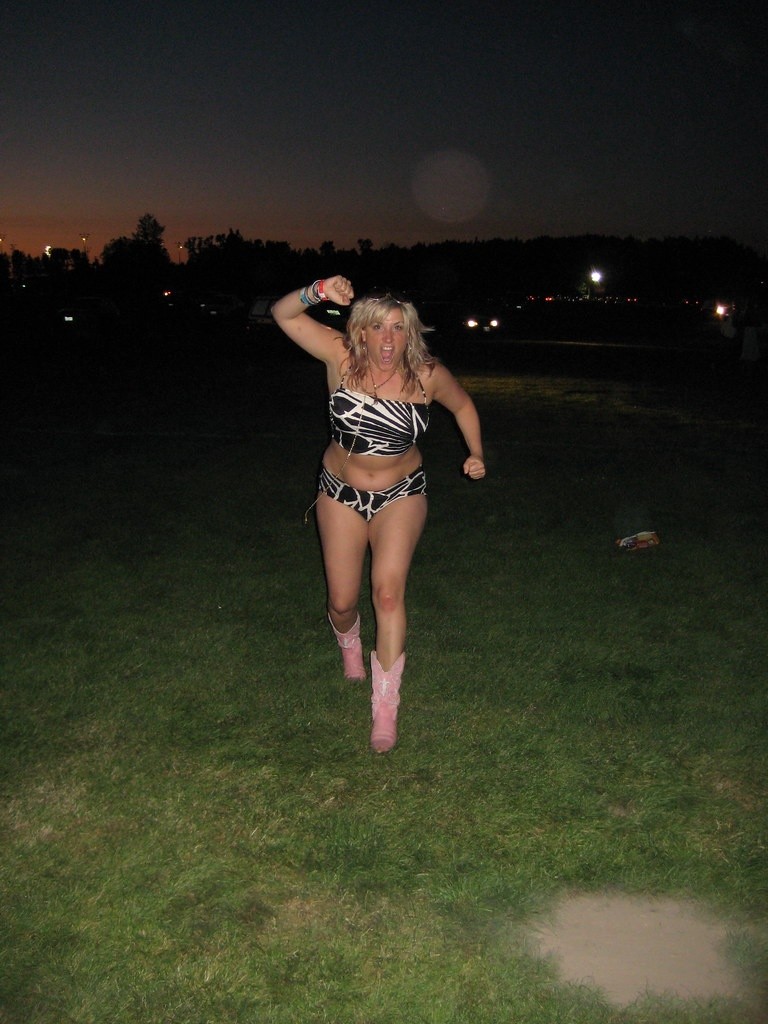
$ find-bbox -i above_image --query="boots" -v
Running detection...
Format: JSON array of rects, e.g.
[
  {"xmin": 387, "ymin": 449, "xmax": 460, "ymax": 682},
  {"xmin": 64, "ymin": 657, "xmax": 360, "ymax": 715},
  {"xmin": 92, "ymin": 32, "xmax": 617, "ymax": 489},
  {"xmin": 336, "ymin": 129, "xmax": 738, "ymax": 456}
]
[
  {"xmin": 370, "ymin": 648, "xmax": 405, "ymax": 754},
  {"xmin": 327, "ymin": 611, "xmax": 367, "ymax": 680}
]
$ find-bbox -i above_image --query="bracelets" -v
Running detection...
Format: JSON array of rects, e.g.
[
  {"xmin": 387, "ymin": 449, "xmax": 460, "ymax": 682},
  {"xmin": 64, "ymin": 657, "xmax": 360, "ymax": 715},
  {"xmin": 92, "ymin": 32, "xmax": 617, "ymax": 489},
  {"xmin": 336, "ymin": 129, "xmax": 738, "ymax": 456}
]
[
  {"xmin": 317, "ymin": 280, "xmax": 329, "ymax": 302},
  {"xmin": 311, "ymin": 279, "xmax": 322, "ymax": 303},
  {"xmin": 300, "ymin": 286, "xmax": 316, "ymax": 306}
]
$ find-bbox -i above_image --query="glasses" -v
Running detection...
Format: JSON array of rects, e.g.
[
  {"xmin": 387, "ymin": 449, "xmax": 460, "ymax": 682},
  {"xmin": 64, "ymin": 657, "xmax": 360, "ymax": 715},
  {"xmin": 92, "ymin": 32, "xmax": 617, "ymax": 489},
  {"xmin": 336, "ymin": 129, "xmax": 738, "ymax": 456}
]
[{"xmin": 365, "ymin": 285, "xmax": 411, "ymax": 304}]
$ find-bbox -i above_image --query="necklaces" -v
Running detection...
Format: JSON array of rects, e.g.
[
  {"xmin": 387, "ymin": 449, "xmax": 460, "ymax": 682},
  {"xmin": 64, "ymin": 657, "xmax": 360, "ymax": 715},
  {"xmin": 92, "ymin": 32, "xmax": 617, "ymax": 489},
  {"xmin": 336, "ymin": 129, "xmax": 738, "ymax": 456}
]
[{"xmin": 368, "ymin": 363, "xmax": 397, "ymax": 405}]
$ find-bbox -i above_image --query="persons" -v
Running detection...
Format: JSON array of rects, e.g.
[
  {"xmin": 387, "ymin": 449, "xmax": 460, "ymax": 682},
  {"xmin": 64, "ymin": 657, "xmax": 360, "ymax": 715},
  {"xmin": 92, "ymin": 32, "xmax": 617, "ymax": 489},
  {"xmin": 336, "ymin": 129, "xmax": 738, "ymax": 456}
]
[{"xmin": 269, "ymin": 275, "xmax": 488, "ymax": 754}]
